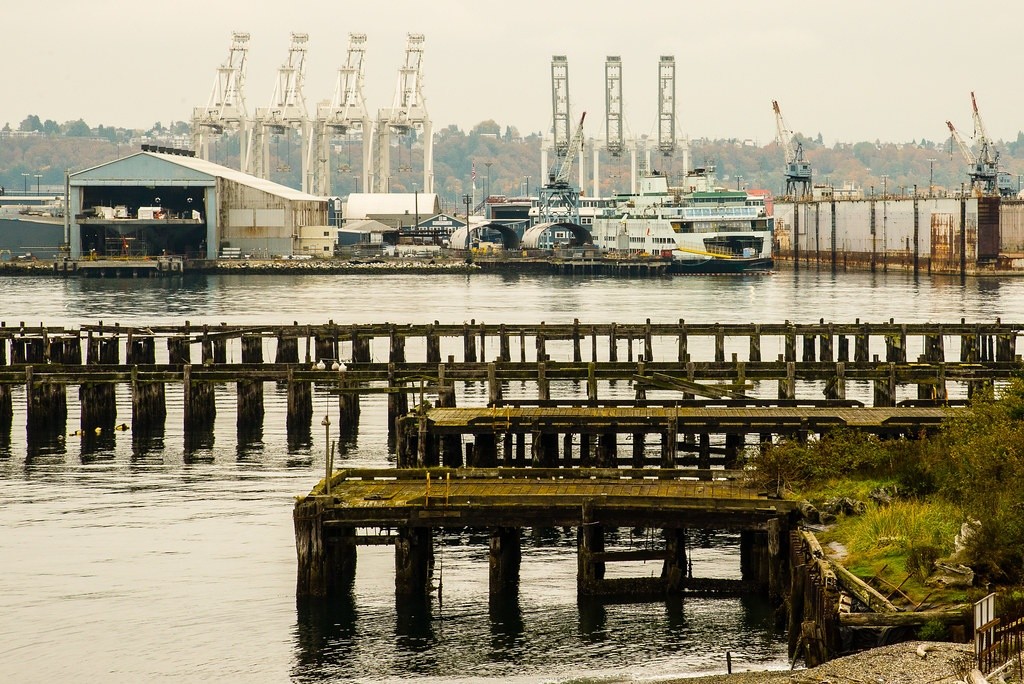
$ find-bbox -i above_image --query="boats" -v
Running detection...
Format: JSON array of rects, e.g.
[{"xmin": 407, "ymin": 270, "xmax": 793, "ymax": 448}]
[{"xmin": 591, "ymin": 167, "xmax": 775, "ymax": 273}]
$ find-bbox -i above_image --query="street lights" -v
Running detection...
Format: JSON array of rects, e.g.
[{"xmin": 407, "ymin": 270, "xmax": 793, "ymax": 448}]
[
  {"xmin": 22, "ymin": 174, "xmax": 29, "ymax": 196},
  {"xmin": 320, "ymin": 158, "xmax": 329, "ymax": 196},
  {"xmin": 429, "ymin": 173, "xmax": 433, "ymax": 192},
  {"xmin": 369, "ymin": 173, "xmax": 374, "ymax": 193},
  {"xmin": 524, "ymin": 175, "xmax": 532, "ymax": 196},
  {"xmin": 480, "ymin": 176, "xmax": 488, "ymax": 206},
  {"xmin": 388, "ymin": 177, "xmax": 392, "ymax": 193},
  {"xmin": 34, "ymin": 175, "xmax": 43, "ymax": 195},
  {"xmin": 486, "ymin": 161, "xmax": 493, "ymax": 195},
  {"xmin": 412, "ymin": 183, "xmax": 417, "ymax": 193},
  {"xmin": 521, "ymin": 183, "xmax": 526, "ymax": 196},
  {"xmin": 497, "ymin": 179, "xmax": 503, "ymax": 195},
  {"xmin": 353, "ymin": 176, "xmax": 359, "ymax": 193}
]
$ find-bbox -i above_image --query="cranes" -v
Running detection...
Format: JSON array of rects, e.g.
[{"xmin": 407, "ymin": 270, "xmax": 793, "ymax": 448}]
[
  {"xmin": 945, "ymin": 90, "xmax": 1001, "ymax": 196},
  {"xmin": 538, "ymin": 111, "xmax": 588, "ymax": 248},
  {"xmin": 771, "ymin": 98, "xmax": 813, "ymax": 201}
]
